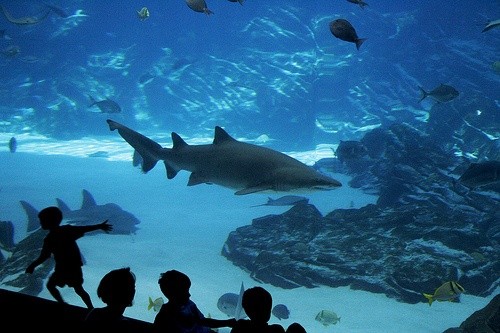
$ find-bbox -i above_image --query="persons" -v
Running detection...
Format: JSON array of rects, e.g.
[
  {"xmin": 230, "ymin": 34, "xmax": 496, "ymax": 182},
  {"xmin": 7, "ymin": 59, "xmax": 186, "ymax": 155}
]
[
  {"xmin": 80, "ymin": 267, "xmax": 140, "ymax": 333},
  {"xmin": 150, "ymin": 269, "xmax": 238, "ymax": 333},
  {"xmin": 230, "ymin": 286, "xmax": 286, "ymax": 333},
  {"xmin": 23, "ymin": 206, "xmax": 114, "ymax": 310}
]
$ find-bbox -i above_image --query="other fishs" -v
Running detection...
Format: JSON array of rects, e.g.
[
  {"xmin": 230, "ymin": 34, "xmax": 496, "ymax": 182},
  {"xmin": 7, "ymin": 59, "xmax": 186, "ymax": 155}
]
[
  {"xmin": 147, "ymin": 296, "xmax": 164, "ymax": 313},
  {"xmin": 135, "ymin": 6, "xmax": 150, "ymax": 21},
  {"xmin": 0, "ymin": 0, "xmax": 70, "ymax": 64},
  {"xmin": 185, "ymin": 0, "xmax": 215, "ymax": 16},
  {"xmin": 272, "ymin": 304, "xmax": 290, "ymax": 321},
  {"xmin": 138, "ymin": 71, "xmax": 155, "ymax": 85},
  {"xmin": 458, "ymin": 160, "xmax": 500, "ymax": 187},
  {"xmin": 86, "ymin": 96, "xmax": 121, "ymax": 114},
  {"xmin": 328, "ymin": 19, "xmax": 367, "ymax": 50},
  {"xmin": 346, "ymin": 0, "xmax": 369, "ymax": 9},
  {"xmin": 314, "ymin": 310, "xmax": 341, "ymax": 327},
  {"xmin": 334, "ymin": 139, "xmax": 368, "ymax": 157},
  {"xmin": 265, "ymin": 195, "xmax": 309, "ymax": 205},
  {"xmin": 217, "ymin": 282, "xmax": 248, "ymax": 321},
  {"xmin": 422, "ymin": 281, "xmax": 465, "ymax": 307},
  {"xmin": 416, "ymin": 82, "xmax": 460, "ymax": 105}
]
